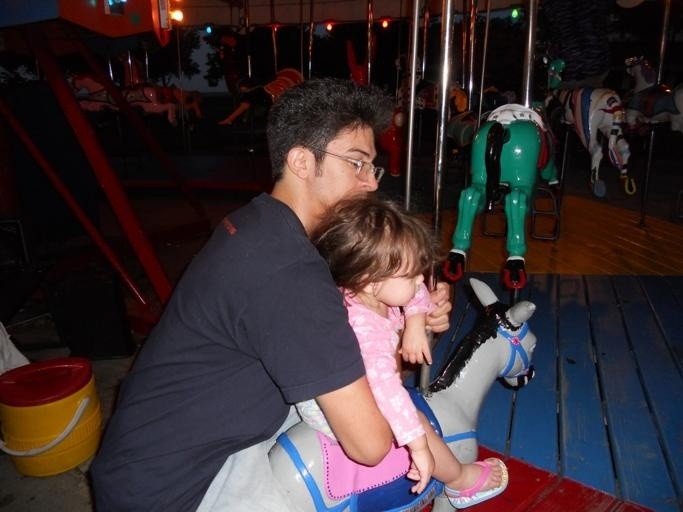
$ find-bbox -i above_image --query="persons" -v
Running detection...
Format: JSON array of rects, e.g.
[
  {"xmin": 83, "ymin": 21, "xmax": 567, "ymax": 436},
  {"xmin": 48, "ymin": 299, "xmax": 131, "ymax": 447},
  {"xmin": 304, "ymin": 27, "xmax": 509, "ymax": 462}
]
[
  {"xmin": 77, "ymin": 53, "xmax": 681, "ymax": 199},
  {"xmin": 88, "ymin": 80, "xmax": 454, "ymax": 511},
  {"xmin": 292, "ymin": 193, "xmax": 509, "ymax": 509}
]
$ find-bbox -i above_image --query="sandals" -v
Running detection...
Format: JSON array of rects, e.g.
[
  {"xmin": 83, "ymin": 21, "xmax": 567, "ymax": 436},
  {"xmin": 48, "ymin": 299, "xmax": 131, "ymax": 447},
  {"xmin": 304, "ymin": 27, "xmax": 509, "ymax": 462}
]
[{"xmin": 442, "ymin": 458, "xmax": 509, "ymax": 510}]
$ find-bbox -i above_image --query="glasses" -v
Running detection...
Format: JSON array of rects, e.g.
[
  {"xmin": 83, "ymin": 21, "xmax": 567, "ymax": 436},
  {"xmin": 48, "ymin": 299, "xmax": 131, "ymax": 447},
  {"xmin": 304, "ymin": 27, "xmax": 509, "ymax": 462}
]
[{"xmin": 306, "ymin": 145, "xmax": 386, "ymax": 185}]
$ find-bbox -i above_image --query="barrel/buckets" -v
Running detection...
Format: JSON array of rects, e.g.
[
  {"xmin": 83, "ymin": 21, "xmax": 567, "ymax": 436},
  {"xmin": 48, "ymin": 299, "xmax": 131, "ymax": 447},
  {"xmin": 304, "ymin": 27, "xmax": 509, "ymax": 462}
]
[{"xmin": 0, "ymin": 358, "xmax": 101, "ymax": 478}]
